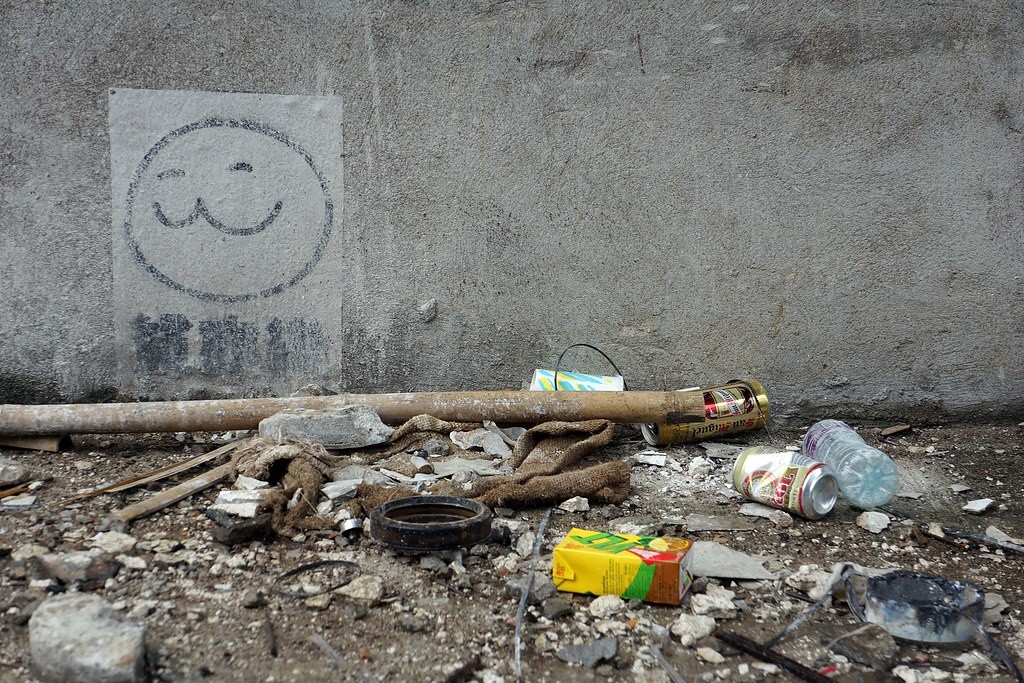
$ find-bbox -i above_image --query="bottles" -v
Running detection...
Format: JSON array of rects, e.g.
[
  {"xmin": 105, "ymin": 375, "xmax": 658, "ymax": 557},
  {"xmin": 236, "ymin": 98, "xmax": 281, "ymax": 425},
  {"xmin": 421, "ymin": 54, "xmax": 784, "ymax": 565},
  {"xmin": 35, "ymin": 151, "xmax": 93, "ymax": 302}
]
[{"xmin": 802, "ymin": 419, "xmax": 899, "ymax": 510}]
